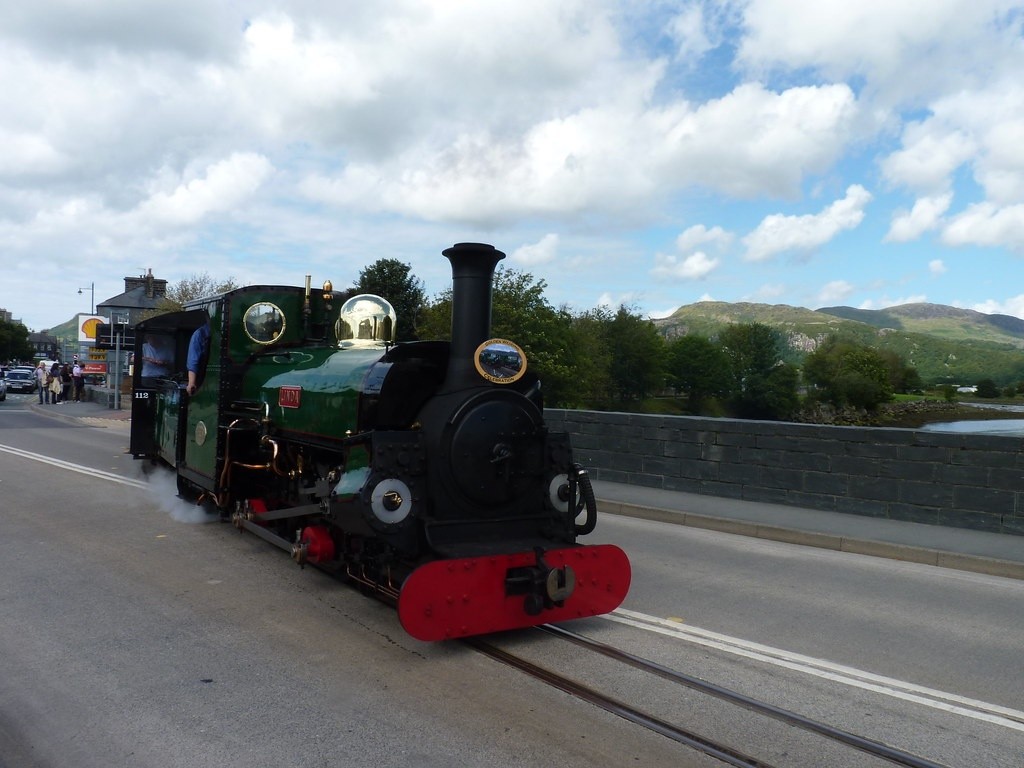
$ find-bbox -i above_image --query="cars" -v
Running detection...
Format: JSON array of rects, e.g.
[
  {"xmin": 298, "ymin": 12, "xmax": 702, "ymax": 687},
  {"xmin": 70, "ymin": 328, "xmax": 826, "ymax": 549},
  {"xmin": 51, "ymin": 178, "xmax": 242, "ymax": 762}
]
[
  {"xmin": 0, "ymin": 369, "xmax": 7, "ymax": 402},
  {"xmin": 4, "ymin": 368, "xmax": 38, "ymax": 394},
  {"xmin": 11, "ymin": 356, "xmax": 49, "ymax": 390}
]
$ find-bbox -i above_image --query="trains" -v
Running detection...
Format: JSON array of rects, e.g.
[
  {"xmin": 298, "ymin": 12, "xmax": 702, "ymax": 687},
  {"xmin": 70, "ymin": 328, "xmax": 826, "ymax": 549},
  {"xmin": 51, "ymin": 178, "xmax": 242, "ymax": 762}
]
[{"xmin": 130, "ymin": 242, "xmax": 633, "ymax": 641}]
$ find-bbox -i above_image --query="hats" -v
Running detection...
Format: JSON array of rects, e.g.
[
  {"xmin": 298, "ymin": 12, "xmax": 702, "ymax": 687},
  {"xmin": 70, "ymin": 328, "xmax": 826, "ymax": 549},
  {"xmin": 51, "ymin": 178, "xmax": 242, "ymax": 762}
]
[
  {"xmin": 63, "ymin": 362, "xmax": 68, "ymax": 364},
  {"xmin": 39, "ymin": 362, "xmax": 45, "ymax": 368}
]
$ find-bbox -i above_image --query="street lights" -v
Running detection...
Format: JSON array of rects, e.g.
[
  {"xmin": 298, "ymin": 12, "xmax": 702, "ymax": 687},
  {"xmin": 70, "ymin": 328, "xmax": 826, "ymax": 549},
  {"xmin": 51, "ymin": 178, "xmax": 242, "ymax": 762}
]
[{"xmin": 78, "ymin": 282, "xmax": 94, "ymax": 315}]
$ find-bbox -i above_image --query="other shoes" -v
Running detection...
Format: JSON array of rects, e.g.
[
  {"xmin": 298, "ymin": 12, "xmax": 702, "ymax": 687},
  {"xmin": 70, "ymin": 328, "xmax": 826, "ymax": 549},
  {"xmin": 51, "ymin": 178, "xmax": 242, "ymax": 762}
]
[
  {"xmin": 39, "ymin": 402, "xmax": 42, "ymax": 404},
  {"xmin": 46, "ymin": 402, "xmax": 50, "ymax": 404}
]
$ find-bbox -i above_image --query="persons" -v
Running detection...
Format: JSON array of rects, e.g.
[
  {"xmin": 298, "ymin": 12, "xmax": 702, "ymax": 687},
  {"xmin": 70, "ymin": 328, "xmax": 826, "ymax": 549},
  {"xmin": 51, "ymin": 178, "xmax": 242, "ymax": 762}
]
[
  {"xmin": 60, "ymin": 362, "xmax": 72, "ymax": 402},
  {"xmin": 51, "ymin": 364, "xmax": 64, "ymax": 405},
  {"xmin": 34, "ymin": 362, "xmax": 50, "ymax": 405},
  {"xmin": 131, "ymin": 334, "xmax": 174, "ymax": 377},
  {"xmin": 186, "ymin": 323, "xmax": 209, "ymax": 397},
  {"xmin": 74, "ymin": 363, "xmax": 85, "ymax": 403}
]
